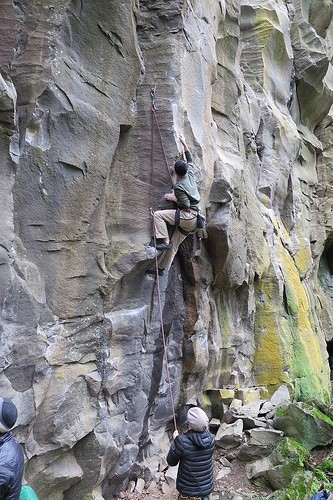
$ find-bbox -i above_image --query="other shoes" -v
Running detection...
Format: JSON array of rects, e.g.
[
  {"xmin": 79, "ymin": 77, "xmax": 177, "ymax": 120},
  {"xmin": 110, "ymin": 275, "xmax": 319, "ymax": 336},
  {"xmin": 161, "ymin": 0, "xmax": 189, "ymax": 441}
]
[
  {"xmin": 153, "ymin": 240, "xmax": 173, "ymax": 249},
  {"xmin": 145, "ymin": 267, "xmax": 164, "ymax": 276}
]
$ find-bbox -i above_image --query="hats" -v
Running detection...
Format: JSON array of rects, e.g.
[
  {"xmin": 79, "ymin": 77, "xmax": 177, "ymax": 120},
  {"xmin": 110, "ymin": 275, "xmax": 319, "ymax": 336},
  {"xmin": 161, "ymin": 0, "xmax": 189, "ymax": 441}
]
[{"xmin": 0, "ymin": 397, "xmax": 18, "ymax": 431}]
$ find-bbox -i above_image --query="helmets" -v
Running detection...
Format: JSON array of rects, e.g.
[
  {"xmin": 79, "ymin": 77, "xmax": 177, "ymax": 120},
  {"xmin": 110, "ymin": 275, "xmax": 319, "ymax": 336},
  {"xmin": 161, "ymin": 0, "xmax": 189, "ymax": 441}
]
[{"xmin": 187, "ymin": 407, "xmax": 209, "ymax": 432}]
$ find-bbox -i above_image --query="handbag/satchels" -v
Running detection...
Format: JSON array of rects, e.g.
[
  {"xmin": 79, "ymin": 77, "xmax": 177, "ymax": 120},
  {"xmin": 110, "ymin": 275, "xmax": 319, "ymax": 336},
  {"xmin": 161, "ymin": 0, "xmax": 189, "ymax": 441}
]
[{"xmin": 197, "ymin": 214, "xmax": 206, "ymax": 229}]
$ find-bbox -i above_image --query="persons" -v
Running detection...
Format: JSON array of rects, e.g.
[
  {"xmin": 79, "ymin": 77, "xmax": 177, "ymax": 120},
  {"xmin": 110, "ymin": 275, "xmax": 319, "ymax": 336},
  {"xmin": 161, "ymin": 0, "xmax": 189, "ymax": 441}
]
[
  {"xmin": 167, "ymin": 407, "xmax": 214, "ymax": 500},
  {"xmin": 0, "ymin": 398, "xmax": 24, "ymax": 500},
  {"xmin": 146, "ymin": 160, "xmax": 201, "ymax": 276}
]
